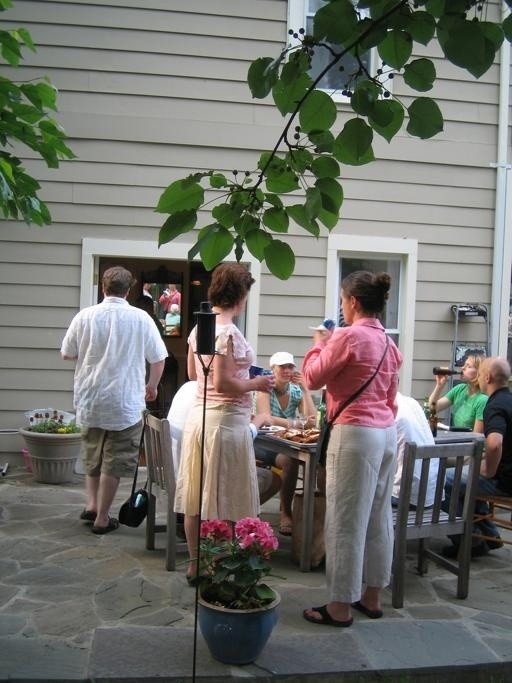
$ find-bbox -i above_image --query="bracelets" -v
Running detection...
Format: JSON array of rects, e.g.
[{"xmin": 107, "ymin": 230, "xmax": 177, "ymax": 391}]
[{"xmin": 292, "ymin": 420, "xmax": 297, "ymax": 428}]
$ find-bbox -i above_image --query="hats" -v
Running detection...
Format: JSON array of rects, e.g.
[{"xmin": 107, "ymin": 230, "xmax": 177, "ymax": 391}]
[{"xmin": 270, "ymin": 351, "xmax": 295, "ymax": 369}]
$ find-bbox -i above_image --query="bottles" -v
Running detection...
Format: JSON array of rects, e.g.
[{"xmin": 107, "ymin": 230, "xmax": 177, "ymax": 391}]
[
  {"xmin": 429, "ymin": 403, "xmax": 437, "ymax": 436},
  {"xmin": 432, "ymin": 367, "xmax": 462, "ymax": 376},
  {"xmin": 316, "ymin": 389, "xmax": 326, "ymax": 430},
  {"xmin": 424, "ymin": 394, "xmax": 430, "ymax": 420}
]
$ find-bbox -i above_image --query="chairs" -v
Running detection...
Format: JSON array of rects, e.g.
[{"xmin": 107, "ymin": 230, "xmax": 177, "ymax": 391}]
[
  {"xmin": 473, "ymin": 494, "xmax": 512, "ymax": 545},
  {"xmin": 141, "ymin": 409, "xmax": 187, "ymax": 571},
  {"xmin": 392, "ymin": 437, "xmax": 484, "ymax": 608}
]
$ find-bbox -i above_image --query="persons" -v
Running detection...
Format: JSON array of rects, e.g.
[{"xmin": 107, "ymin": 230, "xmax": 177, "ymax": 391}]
[
  {"xmin": 386, "ymin": 377, "xmax": 447, "ymax": 512},
  {"xmin": 442, "ymin": 357, "xmax": 512, "ymax": 560},
  {"xmin": 61, "ymin": 266, "xmax": 169, "ymax": 535},
  {"xmin": 429, "ymin": 353, "xmax": 488, "ymax": 466},
  {"xmin": 165, "ymin": 378, "xmax": 282, "ymax": 515},
  {"xmin": 252, "ymin": 351, "xmax": 316, "ymax": 535},
  {"xmin": 170, "ymin": 323, "xmax": 181, "ymax": 335},
  {"xmin": 143, "ymin": 282, "xmax": 180, "ymax": 336},
  {"xmin": 133, "ymin": 297, "xmax": 165, "ymax": 428},
  {"xmin": 174, "ymin": 265, "xmax": 276, "ymax": 588},
  {"xmin": 303, "ymin": 269, "xmax": 403, "ymax": 627}
]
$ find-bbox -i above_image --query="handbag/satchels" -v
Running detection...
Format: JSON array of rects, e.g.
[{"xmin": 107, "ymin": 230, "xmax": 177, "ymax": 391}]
[
  {"xmin": 288, "ymin": 491, "xmax": 331, "ymax": 570},
  {"xmin": 119, "ymin": 489, "xmax": 148, "ymax": 528},
  {"xmin": 316, "ymin": 420, "xmax": 330, "ymax": 468}
]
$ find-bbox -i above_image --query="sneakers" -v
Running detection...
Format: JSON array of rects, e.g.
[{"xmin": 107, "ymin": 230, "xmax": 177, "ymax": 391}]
[
  {"xmin": 442, "ymin": 540, "xmax": 490, "ymax": 559},
  {"xmin": 484, "ymin": 534, "xmax": 504, "ymax": 550}
]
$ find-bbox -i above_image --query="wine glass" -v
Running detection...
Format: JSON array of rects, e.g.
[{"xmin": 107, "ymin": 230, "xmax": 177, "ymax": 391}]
[{"xmin": 298, "ymin": 413, "xmax": 309, "ymax": 432}]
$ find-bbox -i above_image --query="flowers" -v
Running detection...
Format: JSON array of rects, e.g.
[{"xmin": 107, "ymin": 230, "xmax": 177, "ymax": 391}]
[
  {"xmin": 27, "ymin": 409, "xmax": 81, "ymax": 433},
  {"xmin": 175, "ymin": 518, "xmax": 287, "ymax": 602}
]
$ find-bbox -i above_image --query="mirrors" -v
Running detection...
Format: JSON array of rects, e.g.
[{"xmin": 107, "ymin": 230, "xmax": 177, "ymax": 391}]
[{"xmin": 141, "ymin": 281, "xmax": 182, "ymax": 337}]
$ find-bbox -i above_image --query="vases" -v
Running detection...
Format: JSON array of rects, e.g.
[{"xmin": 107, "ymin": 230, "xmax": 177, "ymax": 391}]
[
  {"xmin": 19, "ymin": 425, "xmax": 81, "ymax": 484},
  {"xmin": 197, "ymin": 584, "xmax": 281, "ymax": 664}
]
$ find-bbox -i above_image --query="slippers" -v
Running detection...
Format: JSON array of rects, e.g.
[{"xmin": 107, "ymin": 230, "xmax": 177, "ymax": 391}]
[
  {"xmin": 79, "ymin": 510, "xmax": 95, "ymax": 521},
  {"xmin": 303, "ymin": 604, "xmax": 353, "ymax": 627},
  {"xmin": 186, "ymin": 576, "xmax": 211, "ymax": 587},
  {"xmin": 279, "ymin": 517, "xmax": 292, "ymax": 536},
  {"xmin": 352, "ymin": 601, "xmax": 383, "ymax": 619},
  {"xmin": 89, "ymin": 518, "xmax": 120, "ymax": 534}
]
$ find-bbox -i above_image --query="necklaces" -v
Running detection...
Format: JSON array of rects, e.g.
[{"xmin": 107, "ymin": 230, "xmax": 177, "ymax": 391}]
[{"xmin": 273, "ymin": 384, "xmax": 290, "ymax": 396}]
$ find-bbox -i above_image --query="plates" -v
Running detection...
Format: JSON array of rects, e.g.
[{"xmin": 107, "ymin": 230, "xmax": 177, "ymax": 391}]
[{"xmin": 257, "ymin": 427, "xmax": 279, "ymax": 434}]
